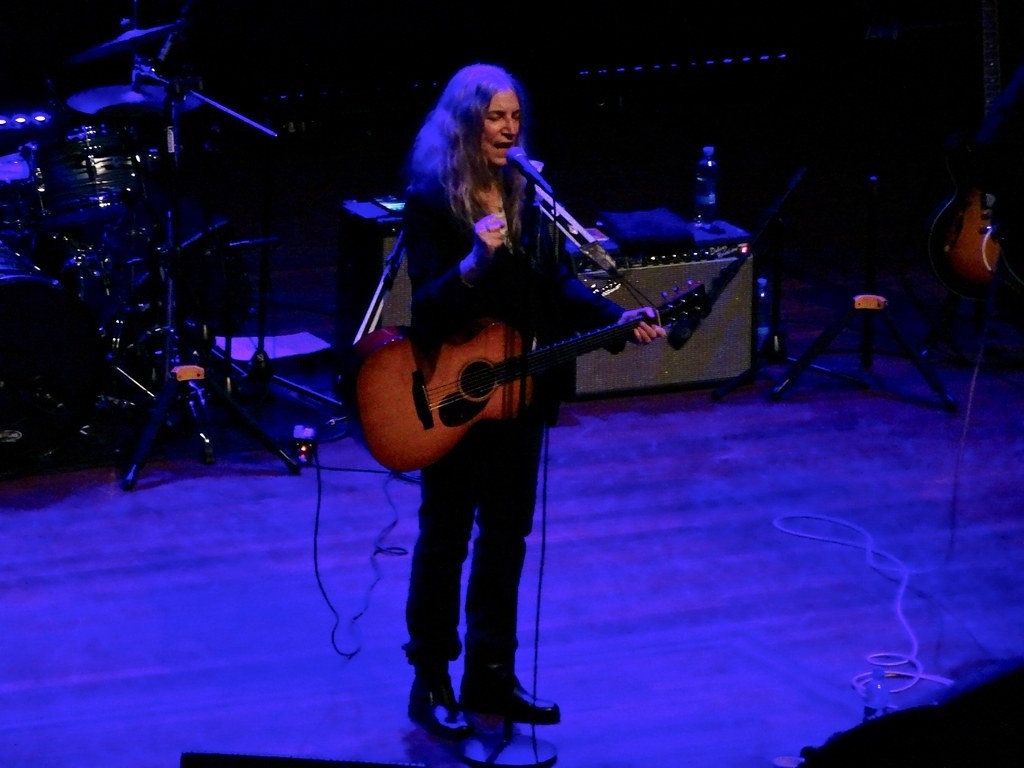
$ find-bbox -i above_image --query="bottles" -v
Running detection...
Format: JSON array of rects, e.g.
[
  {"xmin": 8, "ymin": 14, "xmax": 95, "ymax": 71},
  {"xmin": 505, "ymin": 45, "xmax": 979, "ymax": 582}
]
[
  {"xmin": 750, "ymin": 278, "xmax": 773, "ymax": 352},
  {"xmin": 862, "ymin": 669, "xmax": 894, "ymax": 724},
  {"xmin": 692, "ymin": 145, "xmax": 717, "ymax": 232}
]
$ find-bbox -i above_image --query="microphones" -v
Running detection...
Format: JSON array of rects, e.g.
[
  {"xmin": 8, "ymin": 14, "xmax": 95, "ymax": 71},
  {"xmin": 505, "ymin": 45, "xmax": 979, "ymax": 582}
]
[{"xmin": 506, "ymin": 147, "xmax": 552, "ymax": 195}]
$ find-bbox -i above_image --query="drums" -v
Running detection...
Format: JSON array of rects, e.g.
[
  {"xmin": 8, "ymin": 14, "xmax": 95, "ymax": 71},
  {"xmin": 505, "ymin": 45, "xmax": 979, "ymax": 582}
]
[
  {"xmin": 30, "ymin": 124, "xmax": 152, "ymax": 225},
  {"xmin": 0, "ymin": 250, "xmax": 112, "ymax": 467}
]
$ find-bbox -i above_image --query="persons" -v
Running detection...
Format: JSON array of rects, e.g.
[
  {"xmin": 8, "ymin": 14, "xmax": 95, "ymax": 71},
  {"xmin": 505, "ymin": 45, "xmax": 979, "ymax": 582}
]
[{"xmin": 399, "ymin": 65, "xmax": 668, "ymax": 744}]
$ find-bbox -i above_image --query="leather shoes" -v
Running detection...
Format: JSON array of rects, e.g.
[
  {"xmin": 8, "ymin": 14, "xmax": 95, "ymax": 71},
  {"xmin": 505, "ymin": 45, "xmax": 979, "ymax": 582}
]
[{"xmin": 408, "ymin": 666, "xmax": 562, "ymax": 739}]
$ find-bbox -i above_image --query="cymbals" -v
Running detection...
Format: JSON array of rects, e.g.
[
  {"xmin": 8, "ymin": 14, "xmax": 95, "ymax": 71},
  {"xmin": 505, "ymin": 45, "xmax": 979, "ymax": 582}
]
[
  {"xmin": 66, "ymin": 17, "xmax": 193, "ymax": 65},
  {"xmin": 67, "ymin": 84, "xmax": 202, "ymax": 117}
]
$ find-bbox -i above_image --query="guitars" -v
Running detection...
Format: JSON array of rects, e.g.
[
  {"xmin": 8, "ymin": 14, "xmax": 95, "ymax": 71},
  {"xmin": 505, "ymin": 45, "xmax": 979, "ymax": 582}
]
[
  {"xmin": 928, "ymin": 1, "xmax": 1022, "ymax": 308},
  {"xmin": 336, "ymin": 275, "xmax": 711, "ymax": 472}
]
